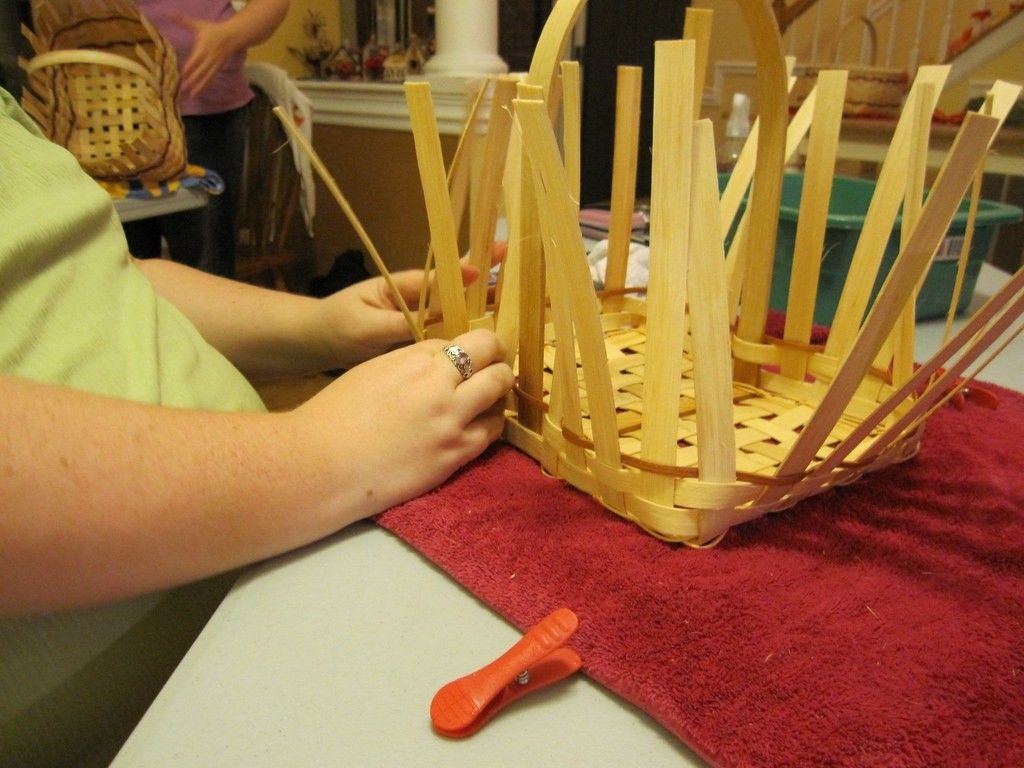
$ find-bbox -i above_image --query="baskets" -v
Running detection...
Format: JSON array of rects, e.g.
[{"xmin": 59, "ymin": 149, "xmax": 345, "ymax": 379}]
[{"xmin": 17, "ymin": 0, "xmax": 186, "ymax": 182}]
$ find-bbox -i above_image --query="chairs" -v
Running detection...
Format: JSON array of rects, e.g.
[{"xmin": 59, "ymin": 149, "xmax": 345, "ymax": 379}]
[{"xmin": 238, "ymin": 60, "xmax": 313, "ymax": 289}]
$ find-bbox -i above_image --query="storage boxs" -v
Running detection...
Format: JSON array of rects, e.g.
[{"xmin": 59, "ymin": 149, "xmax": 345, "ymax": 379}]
[{"xmin": 716, "ymin": 171, "xmax": 1023, "ymax": 325}]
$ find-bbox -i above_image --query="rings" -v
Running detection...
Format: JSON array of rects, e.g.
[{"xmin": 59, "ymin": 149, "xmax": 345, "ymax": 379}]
[{"xmin": 442, "ymin": 343, "xmax": 473, "ymax": 380}]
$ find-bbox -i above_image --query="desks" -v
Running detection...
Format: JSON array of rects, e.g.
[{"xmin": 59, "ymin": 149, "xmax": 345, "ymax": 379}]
[{"xmin": 101, "ymin": 219, "xmax": 1024, "ymax": 768}]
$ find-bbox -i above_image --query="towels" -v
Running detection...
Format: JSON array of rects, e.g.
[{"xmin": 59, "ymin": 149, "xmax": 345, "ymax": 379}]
[
  {"xmin": 95, "ymin": 164, "xmax": 226, "ymax": 201},
  {"xmin": 586, "ymin": 239, "xmax": 650, "ymax": 297},
  {"xmin": 364, "ymin": 308, "xmax": 1024, "ymax": 768}
]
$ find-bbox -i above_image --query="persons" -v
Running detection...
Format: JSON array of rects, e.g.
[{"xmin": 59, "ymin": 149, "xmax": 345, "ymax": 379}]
[{"xmin": 0, "ymin": 0, "xmax": 517, "ymax": 768}]
